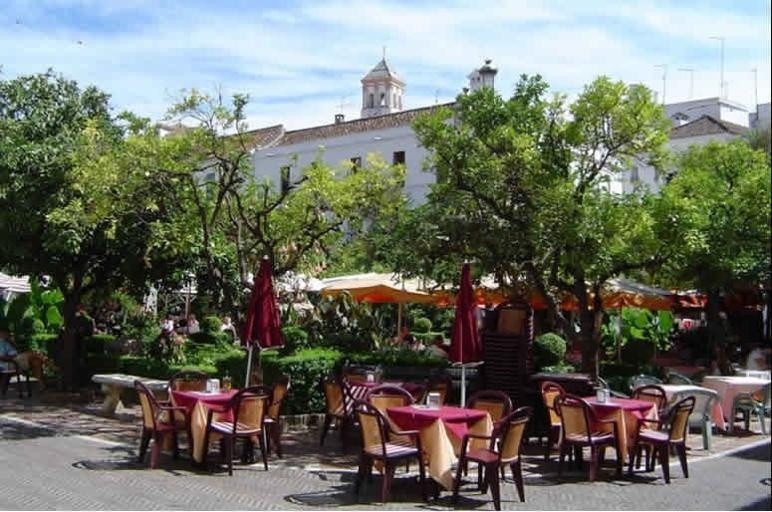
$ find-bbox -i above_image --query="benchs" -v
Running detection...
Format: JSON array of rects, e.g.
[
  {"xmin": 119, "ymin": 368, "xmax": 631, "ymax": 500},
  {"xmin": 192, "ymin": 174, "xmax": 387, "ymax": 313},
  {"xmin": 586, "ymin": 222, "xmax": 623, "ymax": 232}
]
[{"xmin": 91, "ymin": 369, "xmax": 170, "ymax": 415}]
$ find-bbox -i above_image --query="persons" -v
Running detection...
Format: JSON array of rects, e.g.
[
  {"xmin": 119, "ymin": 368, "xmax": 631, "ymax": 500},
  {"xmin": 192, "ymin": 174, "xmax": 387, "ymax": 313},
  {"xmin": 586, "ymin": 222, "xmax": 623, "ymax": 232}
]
[
  {"xmin": 0, "ymin": 324, "xmax": 18, "ymax": 400},
  {"xmin": 670, "ymin": 335, "xmax": 772, "ymax": 420},
  {"xmin": 77, "ymin": 312, "xmax": 235, "ymax": 336}
]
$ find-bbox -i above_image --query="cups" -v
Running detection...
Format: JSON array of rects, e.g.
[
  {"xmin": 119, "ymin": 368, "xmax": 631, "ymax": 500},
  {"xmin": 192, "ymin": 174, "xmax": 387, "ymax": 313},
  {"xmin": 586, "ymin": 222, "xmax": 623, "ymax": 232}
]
[{"xmin": 222, "ymin": 375, "xmax": 232, "ymax": 393}]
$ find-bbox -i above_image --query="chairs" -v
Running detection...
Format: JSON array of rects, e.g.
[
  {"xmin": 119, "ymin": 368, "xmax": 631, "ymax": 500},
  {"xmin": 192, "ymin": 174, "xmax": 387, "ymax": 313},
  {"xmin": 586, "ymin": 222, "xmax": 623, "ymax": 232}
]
[
  {"xmin": 2, "ymin": 354, "xmax": 46, "ymax": 397},
  {"xmin": 320, "ymin": 360, "xmax": 528, "ymax": 512},
  {"xmin": 134, "ymin": 362, "xmax": 291, "ymax": 477},
  {"xmin": 539, "ymin": 356, "xmax": 772, "ymax": 486}
]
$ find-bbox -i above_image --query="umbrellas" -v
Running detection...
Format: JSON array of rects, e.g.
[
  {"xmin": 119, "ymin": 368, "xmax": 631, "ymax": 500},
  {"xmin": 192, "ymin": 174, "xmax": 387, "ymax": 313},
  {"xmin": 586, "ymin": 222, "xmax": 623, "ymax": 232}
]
[
  {"xmin": 241, "ymin": 253, "xmax": 287, "ymax": 389},
  {"xmin": 443, "ymin": 254, "xmax": 488, "ymax": 409}
]
